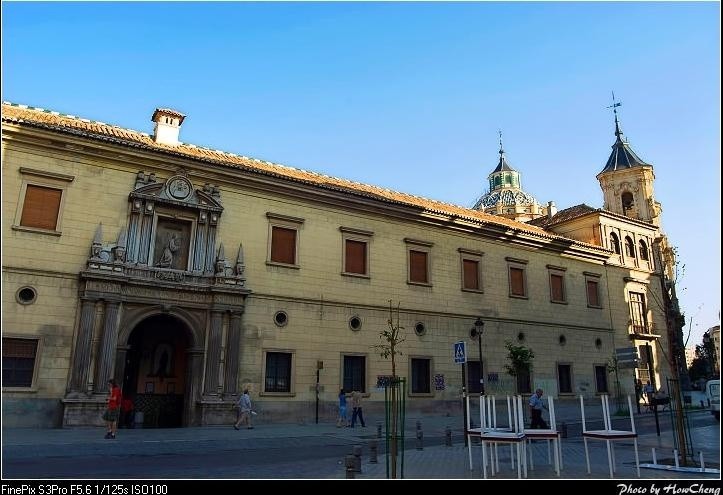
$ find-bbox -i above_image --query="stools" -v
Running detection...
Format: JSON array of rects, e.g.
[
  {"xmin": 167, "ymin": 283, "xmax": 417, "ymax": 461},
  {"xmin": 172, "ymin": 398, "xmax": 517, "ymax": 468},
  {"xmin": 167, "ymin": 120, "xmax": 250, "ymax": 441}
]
[{"xmin": 466, "ymin": 393, "xmax": 643, "ymax": 480}]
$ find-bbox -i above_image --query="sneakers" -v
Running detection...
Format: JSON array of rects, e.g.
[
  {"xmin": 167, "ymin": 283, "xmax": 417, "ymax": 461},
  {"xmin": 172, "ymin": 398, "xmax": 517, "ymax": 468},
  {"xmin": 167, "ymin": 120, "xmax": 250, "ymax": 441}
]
[
  {"xmin": 248, "ymin": 426, "xmax": 254, "ymax": 429},
  {"xmin": 103, "ymin": 431, "xmax": 115, "ymax": 439},
  {"xmin": 233, "ymin": 425, "xmax": 239, "ymax": 430}
]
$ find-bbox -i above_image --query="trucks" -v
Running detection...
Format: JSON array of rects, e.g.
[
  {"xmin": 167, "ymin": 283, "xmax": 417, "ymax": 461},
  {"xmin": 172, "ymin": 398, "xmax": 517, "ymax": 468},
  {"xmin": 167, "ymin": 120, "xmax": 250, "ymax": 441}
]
[{"xmin": 705, "ymin": 380, "xmax": 719, "ymax": 420}]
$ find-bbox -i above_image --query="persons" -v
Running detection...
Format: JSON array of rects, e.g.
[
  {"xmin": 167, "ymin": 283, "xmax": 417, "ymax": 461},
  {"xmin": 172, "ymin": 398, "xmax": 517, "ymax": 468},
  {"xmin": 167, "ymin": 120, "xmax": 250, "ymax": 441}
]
[
  {"xmin": 233, "ymin": 390, "xmax": 255, "ymax": 430},
  {"xmin": 643, "ymin": 380, "xmax": 654, "ymax": 410},
  {"xmin": 159, "ymin": 232, "xmax": 182, "ymax": 267},
  {"xmin": 529, "ymin": 388, "xmax": 549, "ymax": 429},
  {"xmin": 348, "ymin": 387, "xmax": 365, "ymax": 428},
  {"xmin": 637, "ymin": 379, "xmax": 646, "ymax": 403},
  {"xmin": 101, "ymin": 378, "xmax": 122, "ymax": 439},
  {"xmin": 337, "ymin": 389, "xmax": 351, "ymax": 427}
]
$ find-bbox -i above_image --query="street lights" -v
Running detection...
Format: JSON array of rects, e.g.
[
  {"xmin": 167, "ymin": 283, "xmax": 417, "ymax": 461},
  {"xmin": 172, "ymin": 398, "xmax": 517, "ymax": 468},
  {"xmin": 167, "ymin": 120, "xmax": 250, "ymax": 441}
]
[
  {"xmin": 703, "ymin": 331, "xmax": 715, "ymax": 379},
  {"xmin": 474, "ymin": 318, "xmax": 485, "ymax": 397}
]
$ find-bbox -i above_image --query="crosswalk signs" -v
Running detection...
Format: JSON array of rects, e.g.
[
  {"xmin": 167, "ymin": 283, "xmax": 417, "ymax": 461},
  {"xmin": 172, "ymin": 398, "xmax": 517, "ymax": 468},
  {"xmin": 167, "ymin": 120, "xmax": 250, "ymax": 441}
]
[{"xmin": 454, "ymin": 343, "xmax": 467, "ymax": 363}]
